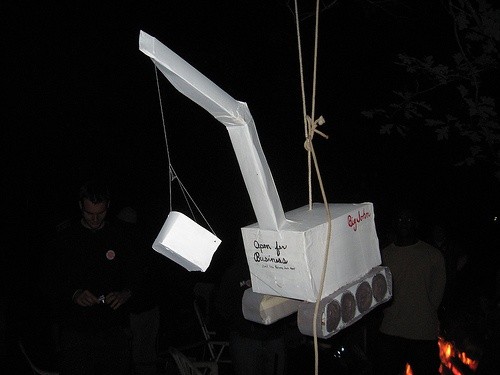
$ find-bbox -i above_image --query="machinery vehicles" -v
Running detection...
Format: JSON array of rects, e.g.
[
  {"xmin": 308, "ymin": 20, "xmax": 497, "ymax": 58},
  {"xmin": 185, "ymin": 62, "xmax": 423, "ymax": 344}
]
[{"xmin": 137, "ymin": 30, "xmax": 393, "ymax": 340}]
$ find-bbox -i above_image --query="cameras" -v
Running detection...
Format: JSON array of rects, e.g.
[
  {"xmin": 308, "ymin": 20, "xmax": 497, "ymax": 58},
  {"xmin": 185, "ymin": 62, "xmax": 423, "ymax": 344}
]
[{"xmin": 97, "ymin": 295, "xmax": 106, "ymax": 303}]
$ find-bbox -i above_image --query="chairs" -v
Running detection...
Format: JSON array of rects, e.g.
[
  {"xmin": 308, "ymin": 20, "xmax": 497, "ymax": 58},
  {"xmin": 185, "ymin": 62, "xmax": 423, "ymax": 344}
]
[{"xmin": 155, "ymin": 295, "xmax": 232, "ymax": 375}]
